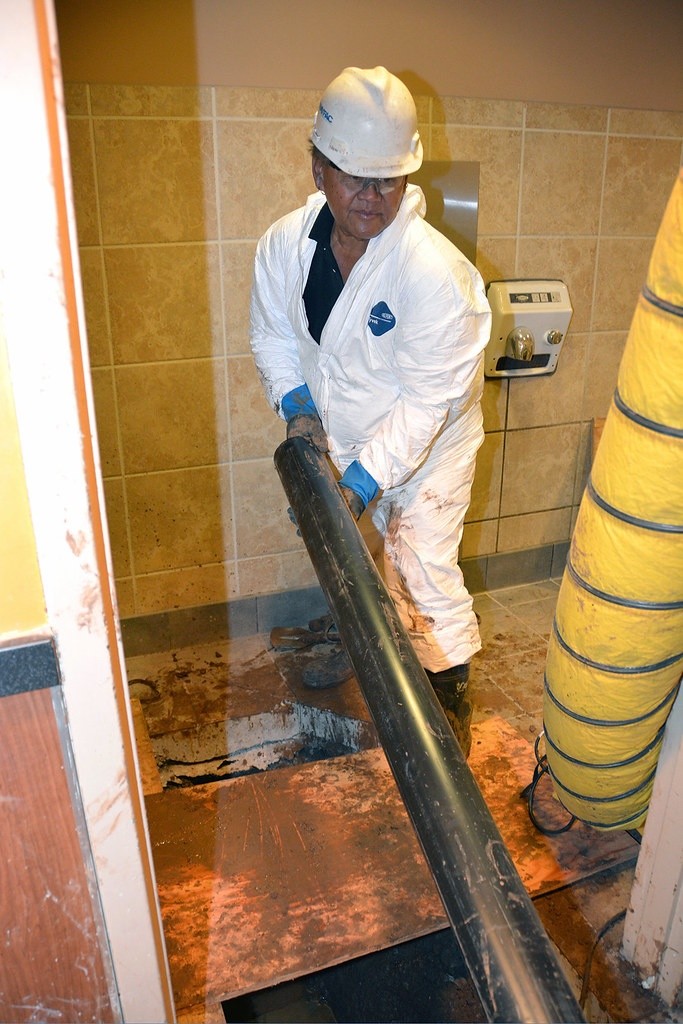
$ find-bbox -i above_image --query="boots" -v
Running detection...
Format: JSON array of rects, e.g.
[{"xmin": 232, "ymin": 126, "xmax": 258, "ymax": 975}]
[
  {"xmin": 426, "ymin": 663, "xmax": 472, "ymax": 764},
  {"xmin": 300, "ymin": 643, "xmax": 355, "ymax": 689}
]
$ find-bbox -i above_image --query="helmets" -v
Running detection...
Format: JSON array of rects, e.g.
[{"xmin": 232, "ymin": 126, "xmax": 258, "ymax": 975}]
[{"xmin": 309, "ymin": 66, "xmax": 424, "ymax": 179}]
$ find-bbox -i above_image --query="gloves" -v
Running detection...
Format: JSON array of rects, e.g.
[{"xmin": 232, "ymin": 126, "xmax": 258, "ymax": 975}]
[
  {"xmin": 287, "ymin": 459, "xmax": 380, "ymax": 537},
  {"xmin": 281, "ymin": 383, "xmax": 328, "ymax": 463}
]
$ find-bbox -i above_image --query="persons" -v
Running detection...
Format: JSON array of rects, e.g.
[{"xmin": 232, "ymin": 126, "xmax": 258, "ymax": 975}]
[{"xmin": 249, "ymin": 65, "xmax": 492, "ymax": 762}]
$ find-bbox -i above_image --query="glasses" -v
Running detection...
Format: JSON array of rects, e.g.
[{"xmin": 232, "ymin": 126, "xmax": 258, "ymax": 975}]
[{"xmin": 317, "ymin": 156, "xmax": 407, "ymax": 196}]
